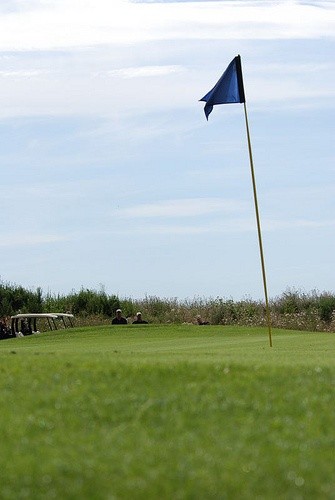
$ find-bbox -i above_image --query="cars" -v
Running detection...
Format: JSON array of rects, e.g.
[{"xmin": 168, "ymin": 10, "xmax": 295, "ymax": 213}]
[{"xmin": 0, "ymin": 313, "xmax": 75, "ymax": 340}]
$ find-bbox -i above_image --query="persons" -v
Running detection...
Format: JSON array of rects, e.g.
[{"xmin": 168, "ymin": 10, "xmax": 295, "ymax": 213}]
[
  {"xmin": 131, "ymin": 312, "xmax": 148, "ymax": 324},
  {"xmin": 111, "ymin": 309, "xmax": 127, "ymax": 325},
  {"xmin": 196, "ymin": 315, "xmax": 211, "ymax": 325}
]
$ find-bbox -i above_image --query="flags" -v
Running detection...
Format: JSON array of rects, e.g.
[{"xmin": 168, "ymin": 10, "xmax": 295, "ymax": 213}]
[{"xmin": 197, "ymin": 56, "xmax": 244, "ymax": 122}]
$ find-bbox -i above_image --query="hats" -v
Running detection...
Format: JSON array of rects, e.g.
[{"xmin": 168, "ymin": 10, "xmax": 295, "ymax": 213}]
[
  {"xmin": 137, "ymin": 312, "xmax": 142, "ymax": 316},
  {"xmin": 116, "ymin": 309, "xmax": 121, "ymax": 313}
]
